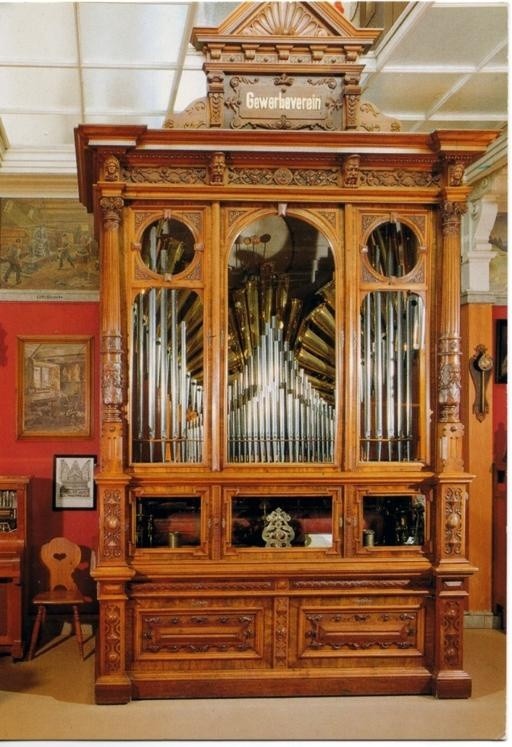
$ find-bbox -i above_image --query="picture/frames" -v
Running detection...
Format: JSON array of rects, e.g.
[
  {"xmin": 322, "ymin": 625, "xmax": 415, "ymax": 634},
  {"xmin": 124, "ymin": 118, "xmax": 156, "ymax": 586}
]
[
  {"xmin": 13, "ymin": 333, "xmax": 96, "ymax": 442},
  {"xmin": 51, "ymin": 453, "xmax": 98, "ymax": 509}
]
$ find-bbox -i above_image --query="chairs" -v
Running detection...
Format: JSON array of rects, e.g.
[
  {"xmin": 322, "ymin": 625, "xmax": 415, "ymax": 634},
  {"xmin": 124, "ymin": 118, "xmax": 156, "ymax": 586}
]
[{"xmin": 25, "ymin": 536, "xmax": 94, "ymax": 664}]
